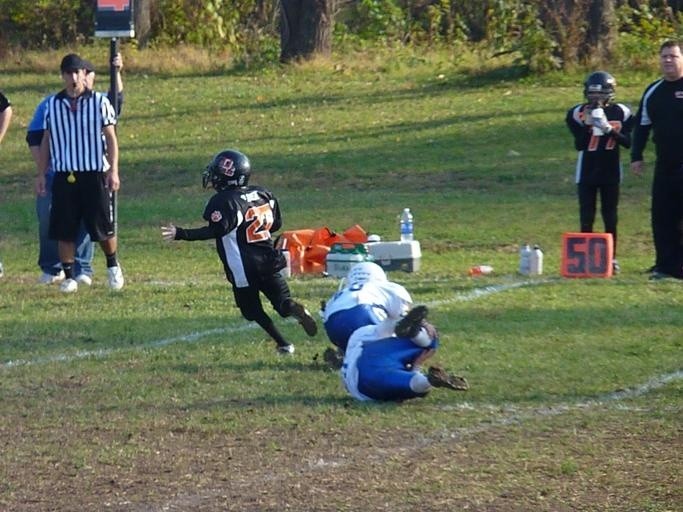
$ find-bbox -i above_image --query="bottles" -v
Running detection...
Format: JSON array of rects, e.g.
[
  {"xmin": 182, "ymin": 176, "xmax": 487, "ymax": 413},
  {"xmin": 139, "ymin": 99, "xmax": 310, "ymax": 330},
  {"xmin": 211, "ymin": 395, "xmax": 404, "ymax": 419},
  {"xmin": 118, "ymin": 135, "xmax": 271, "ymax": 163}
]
[
  {"xmin": 467, "ymin": 266, "xmax": 494, "ymax": 276},
  {"xmin": 520, "ymin": 243, "xmax": 543, "ymax": 275},
  {"xmin": 399, "ymin": 208, "xmax": 413, "ymax": 241}
]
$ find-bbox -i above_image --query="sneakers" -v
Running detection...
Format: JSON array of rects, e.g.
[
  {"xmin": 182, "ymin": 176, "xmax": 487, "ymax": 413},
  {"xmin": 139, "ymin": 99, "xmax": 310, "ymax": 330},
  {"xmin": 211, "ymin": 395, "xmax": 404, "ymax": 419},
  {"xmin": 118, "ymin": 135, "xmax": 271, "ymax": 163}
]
[
  {"xmin": 74, "ymin": 273, "xmax": 92, "ymax": 286},
  {"xmin": 428, "ymin": 365, "xmax": 468, "ymax": 391},
  {"xmin": 394, "ymin": 306, "xmax": 428, "ymax": 337},
  {"xmin": 106, "ymin": 261, "xmax": 124, "ymax": 289},
  {"xmin": 640, "ymin": 266, "xmax": 683, "ymax": 283},
  {"xmin": 294, "ymin": 304, "xmax": 318, "ymax": 337},
  {"xmin": 324, "ymin": 348, "xmax": 343, "ymax": 369},
  {"xmin": 612, "ymin": 260, "xmax": 619, "ymax": 275},
  {"xmin": 37, "ymin": 269, "xmax": 66, "ymax": 284},
  {"xmin": 275, "ymin": 343, "xmax": 295, "ymax": 354},
  {"xmin": 60, "ymin": 278, "xmax": 78, "ymax": 293}
]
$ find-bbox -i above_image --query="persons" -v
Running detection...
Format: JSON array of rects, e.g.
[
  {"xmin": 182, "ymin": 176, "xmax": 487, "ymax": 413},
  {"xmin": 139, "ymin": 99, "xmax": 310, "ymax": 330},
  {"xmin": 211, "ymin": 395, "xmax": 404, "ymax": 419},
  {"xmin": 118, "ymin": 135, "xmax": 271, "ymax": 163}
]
[
  {"xmin": 565, "ymin": 70, "xmax": 635, "ymax": 277},
  {"xmin": 340, "ymin": 303, "xmax": 470, "ymax": 402},
  {"xmin": 630, "ymin": 40, "xmax": 682, "ymax": 278},
  {"xmin": 160, "ymin": 151, "xmax": 317, "ymax": 353},
  {"xmin": 0, "ymin": 91, "xmax": 13, "ymax": 281},
  {"xmin": 319, "ymin": 260, "xmax": 413, "ymax": 370}
]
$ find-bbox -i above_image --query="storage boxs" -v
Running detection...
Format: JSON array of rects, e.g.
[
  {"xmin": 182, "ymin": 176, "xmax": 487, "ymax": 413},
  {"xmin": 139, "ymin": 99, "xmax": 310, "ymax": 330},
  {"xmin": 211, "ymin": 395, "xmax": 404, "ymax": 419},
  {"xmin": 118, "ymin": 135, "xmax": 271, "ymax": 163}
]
[
  {"xmin": 323, "ymin": 240, "xmax": 372, "ymax": 280},
  {"xmin": 365, "ymin": 239, "xmax": 422, "ymax": 275}
]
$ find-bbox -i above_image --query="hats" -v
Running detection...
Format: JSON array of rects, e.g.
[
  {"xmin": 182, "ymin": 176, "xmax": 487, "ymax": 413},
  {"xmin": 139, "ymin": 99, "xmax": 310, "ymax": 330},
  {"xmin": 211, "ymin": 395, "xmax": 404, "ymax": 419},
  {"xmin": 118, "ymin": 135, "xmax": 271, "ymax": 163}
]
[{"xmin": 60, "ymin": 54, "xmax": 95, "ymax": 71}]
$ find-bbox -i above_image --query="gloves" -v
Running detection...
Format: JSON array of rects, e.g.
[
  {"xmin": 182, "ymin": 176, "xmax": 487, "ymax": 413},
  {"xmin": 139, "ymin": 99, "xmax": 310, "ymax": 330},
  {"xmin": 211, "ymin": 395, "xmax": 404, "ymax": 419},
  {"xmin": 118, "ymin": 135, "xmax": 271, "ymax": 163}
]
[{"xmin": 590, "ymin": 108, "xmax": 613, "ymax": 134}]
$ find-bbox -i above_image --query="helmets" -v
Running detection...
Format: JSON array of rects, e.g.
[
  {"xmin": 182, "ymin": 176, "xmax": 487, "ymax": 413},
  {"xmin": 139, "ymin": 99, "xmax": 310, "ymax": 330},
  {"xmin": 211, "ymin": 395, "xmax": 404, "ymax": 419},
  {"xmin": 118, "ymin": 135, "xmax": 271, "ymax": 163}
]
[
  {"xmin": 201, "ymin": 149, "xmax": 251, "ymax": 188},
  {"xmin": 584, "ymin": 71, "xmax": 616, "ymax": 100},
  {"xmin": 347, "ymin": 261, "xmax": 386, "ymax": 279}
]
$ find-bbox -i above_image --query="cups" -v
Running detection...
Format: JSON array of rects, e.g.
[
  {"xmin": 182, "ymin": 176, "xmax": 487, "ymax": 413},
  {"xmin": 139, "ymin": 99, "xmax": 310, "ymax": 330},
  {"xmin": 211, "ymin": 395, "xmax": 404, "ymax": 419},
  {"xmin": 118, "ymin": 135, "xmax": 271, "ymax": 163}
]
[{"xmin": 590, "ymin": 109, "xmax": 606, "ymax": 136}]
